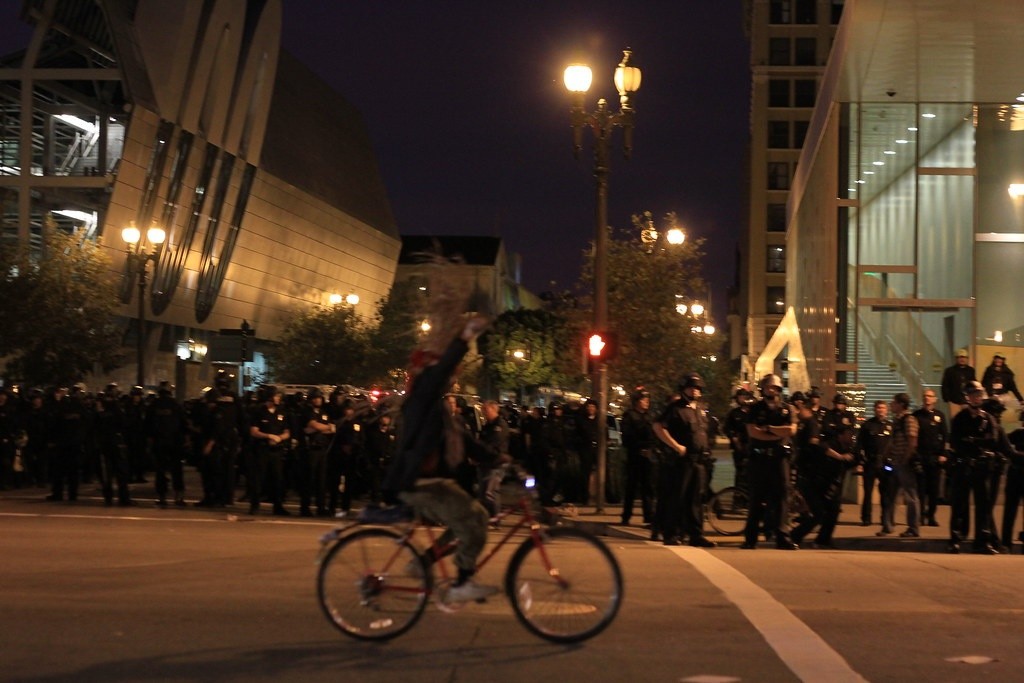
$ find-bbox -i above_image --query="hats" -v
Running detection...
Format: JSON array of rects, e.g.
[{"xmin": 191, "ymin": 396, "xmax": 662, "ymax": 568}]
[
  {"xmin": 26, "ymin": 380, "xmax": 178, "ymax": 401},
  {"xmin": 264, "ymin": 385, "xmax": 347, "ymax": 399},
  {"xmin": 956, "ymin": 349, "xmax": 970, "ymax": 358},
  {"xmin": 993, "ymin": 352, "xmax": 1007, "ymax": 359}
]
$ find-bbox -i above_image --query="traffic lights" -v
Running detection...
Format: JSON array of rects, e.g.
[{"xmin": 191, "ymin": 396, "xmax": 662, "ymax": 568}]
[{"xmin": 588, "ymin": 328, "xmax": 617, "ymax": 362}]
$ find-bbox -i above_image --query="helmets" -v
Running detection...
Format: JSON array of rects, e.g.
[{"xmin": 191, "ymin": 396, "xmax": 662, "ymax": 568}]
[
  {"xmin": 681, "ymin": 373, "xmax": 705, "ymax": 387},
  {"xmin": 215, "ymin": 369, "xmax": 236, "ymax": 386},
  {"xmin": 633, "ymin": 387, "xmax": 651, "ymax": 401},
  {"xmin": 761, "ymin": 374, "xmax": 783, "ymax": 389},
  {"xmin": 832, "ymin": 394, "xmax": 848, "ymax": 404},
  {"xmin": 965, "ymin": 381, "xmax": 986, "ymax": 393}
]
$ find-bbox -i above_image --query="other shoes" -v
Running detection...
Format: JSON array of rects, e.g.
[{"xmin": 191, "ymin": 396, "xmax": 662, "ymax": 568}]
[
  {"xmin": 690, "ymin": 536, "xmax": 718, "ymax": 548},
  {"xmin": 976, "ymin": 542, "xmax": 999, "ymax": 555},
  {"xmin": 948, "ymin": 541, "xmax": 964, "ymax": 553},
  {"xmin": 405, "ymin": 560, "xmax": 435, "ymax": 587},
  {"xmin": 774, "ymin": 534, "xmax": 801, "ymax": 551},
  {"xmin": 900, "ymin": 526, "xmax": 920, "ymax": 538},
  {"xmin": 447, "ymin": 579, "xmax": 501, "ymax": 604},
  {"xmin": 41, "ymin": 494, "xmax": 351, "ymax": 519},
  {"xmin": 875, "ymin": 527, "xmax": 895, "ymax": 537},
  {"xmin": 663, "ymin": 537, "xmax": 682, "ymax": 545},
  {"xmin": 742, "ymin": 538, "xmax": 762, "ymax": 550},
  {"xmin": 921, "ymin": 517, "xmax": 940, "ymax": 527}
]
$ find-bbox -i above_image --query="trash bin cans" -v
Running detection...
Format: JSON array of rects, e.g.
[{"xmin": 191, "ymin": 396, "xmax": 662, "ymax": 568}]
[{"xmin": 595, "ymin": 443, "xmax": 623, "ymax": 502}]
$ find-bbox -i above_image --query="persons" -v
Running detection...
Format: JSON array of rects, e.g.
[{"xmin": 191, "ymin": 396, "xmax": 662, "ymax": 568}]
[
  {"xmin": 0, "ymin": 374, "xmax": 413, "ymax": 517},
  {"xmin": 394, "ymin": 312, "xmax": 511, "ymax": 605},
  {"xmin": 445, "ymin": 383, "xmax": 601, "ymax": 523},
  {"xmin": 856, "ymin": 349, "xmax": 1024, "ymax": 555},
  {"xmin": 620, "ymin": 372, "xmax": 721, "ymax": 546},
  {"xmin": 723, "ymin": 374, "xmax": 857, "ymax": 551}
]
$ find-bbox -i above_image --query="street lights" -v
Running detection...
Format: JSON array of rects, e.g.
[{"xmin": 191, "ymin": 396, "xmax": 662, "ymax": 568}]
[
  {"xmin": 123, "ymin": 225, "xmax": 171, "ymax": 388},
  {"xmin": 561, "ymin": 41, "xmax": 645, "ymax": 511}
]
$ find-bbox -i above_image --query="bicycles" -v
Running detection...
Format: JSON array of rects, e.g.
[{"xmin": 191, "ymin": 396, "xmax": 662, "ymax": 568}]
[{"xmin": 314, "ymin": 471, "xmax": 628, "ymax": 646}]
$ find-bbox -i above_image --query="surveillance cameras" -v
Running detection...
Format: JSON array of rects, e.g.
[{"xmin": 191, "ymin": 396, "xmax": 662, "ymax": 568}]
[{"xmin": 886, "ymin": 91, "xmax": 897, "ymax": 96}]
